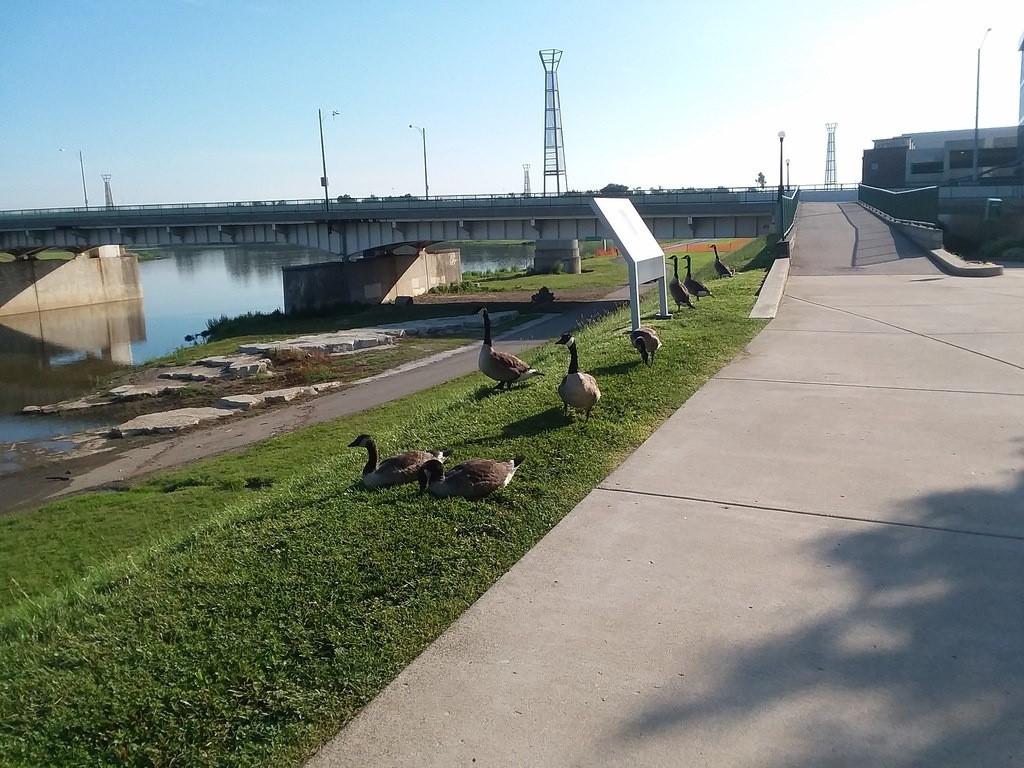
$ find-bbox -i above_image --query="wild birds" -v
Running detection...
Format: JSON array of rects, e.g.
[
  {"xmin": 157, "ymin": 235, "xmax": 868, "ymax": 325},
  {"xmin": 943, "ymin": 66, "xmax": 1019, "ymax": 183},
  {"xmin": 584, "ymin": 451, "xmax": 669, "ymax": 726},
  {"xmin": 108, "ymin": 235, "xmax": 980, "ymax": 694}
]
[
  {"xmin": 348, "ymin": 434, "xmax": 525, "ymax": 503},
  {"xmin": 475, "ymin": 244, "xmax": 735, "ymax": 430}
]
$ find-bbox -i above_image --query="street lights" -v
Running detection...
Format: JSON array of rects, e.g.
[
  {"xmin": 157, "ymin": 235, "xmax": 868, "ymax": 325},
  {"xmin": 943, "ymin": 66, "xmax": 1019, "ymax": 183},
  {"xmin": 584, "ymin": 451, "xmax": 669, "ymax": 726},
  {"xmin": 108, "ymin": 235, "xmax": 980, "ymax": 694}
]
[
  {"xmin": 972, "ymin": 27, "xmax": 992, "ymax": 183},
  {"xmin": 60, "ymin": 148, "xmax": 88, "ymax": 212},
  {"xmin": 786, "ymin": 159, "xmax": 790, "ymax": 192},
  {"xmin": 778, "ymin": 131, "xmax": 785, "ymax": 202},
  {"xmin": 409, "ymin": 124, "xmax": 428, "ymax": 201},
  {"xmin": 319, "ymin": 109, "xmax": 340, "ymax": 213}
]
